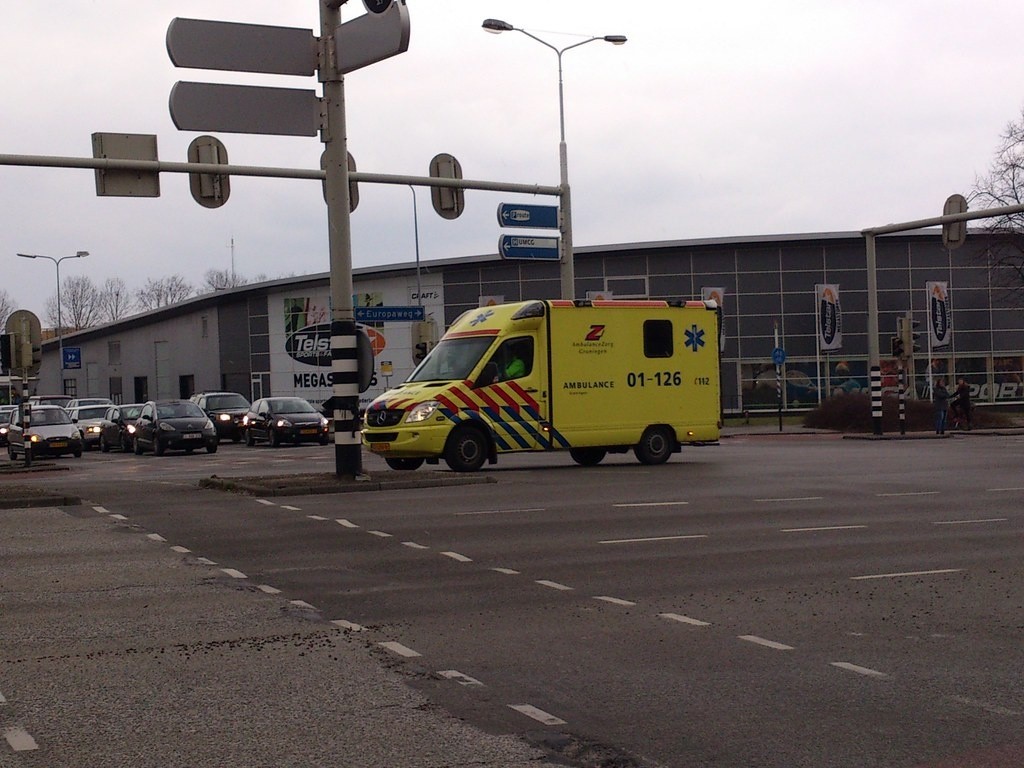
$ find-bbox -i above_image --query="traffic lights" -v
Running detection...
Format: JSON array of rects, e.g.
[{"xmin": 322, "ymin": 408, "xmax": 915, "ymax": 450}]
[
  {"xmin": 913, "ymin": 321, "xmax": 920, "ymax": 352},
  {"xmin": 415, "ymin": 341, "xmax": 429, "ymax": 359},
  {"xmin": 894, "ymin": 339, "xmax": 904, "ymax": 356}
]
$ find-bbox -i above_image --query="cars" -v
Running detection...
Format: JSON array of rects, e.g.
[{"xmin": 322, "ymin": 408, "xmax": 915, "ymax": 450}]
[
  {"xmin": 243, "ymin": 397, "xmax": 329, "ymax": 448},
  {"xmin": 1, "ymin": 410, "xmax": 12, "ymax": 447},
  {"xmin": 28, "ymin": 395, "xmax": 72, "ymax": 408},
  {"xmin": 63, "ymin": 405, "xmax": 119, "ymax": 452},
  {"xmin": 134, "ymin": 399, "xmax": 217, "ymax": 456},
  {"xmin": 65, "ymin": 398, "xmax": 114, "ymax": 409},
  {"xmin": 8, "ymin": 405, "xmax": 82, "ymax": 460},
  {"xmin": 98, "ymin": 403, "xmax": 145, "ymax": 453}
]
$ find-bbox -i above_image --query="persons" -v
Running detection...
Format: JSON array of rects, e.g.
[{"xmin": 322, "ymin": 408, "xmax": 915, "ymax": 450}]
[
  {"xmin": 925, "ymin": 359, "xmax": 975, "ymax": 387},
  {"xmin": 806, "ymin": 362, "xmax": 861, "ymax": 399},
  {"xmin": 492, "ymin": 343, "xmax": 526, "ymax": 383},
  {"xmin": 934, "ymin": 379, "xmax": 950, "ymax": 435},
  {"xmin": 286, "ymin": 298, "xmax": 303, "ymax": 337},
  {"xmin": 950, "ymin": 377, "xmax": 973, "ymax": 431}
]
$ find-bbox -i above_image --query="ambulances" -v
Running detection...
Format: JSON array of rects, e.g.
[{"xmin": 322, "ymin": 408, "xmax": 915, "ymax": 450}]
[{"xmin": 361, "ymin": 298, "xmax": 722, "ymax": 474}]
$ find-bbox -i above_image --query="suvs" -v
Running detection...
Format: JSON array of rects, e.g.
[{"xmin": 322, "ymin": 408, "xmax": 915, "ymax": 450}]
[{"xmin": 189, "ymin": 390, "xmax": 251, "ymax": 442}]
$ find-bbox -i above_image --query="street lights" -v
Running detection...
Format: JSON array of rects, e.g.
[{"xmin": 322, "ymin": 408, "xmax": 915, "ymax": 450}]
[
  {"xmin": 16, "ymin": 251, "xmax": 89, "ymax": 395},
  {"xmin": 482, "ymin": 18, "xmax": 628, "ymax": 300}
]
